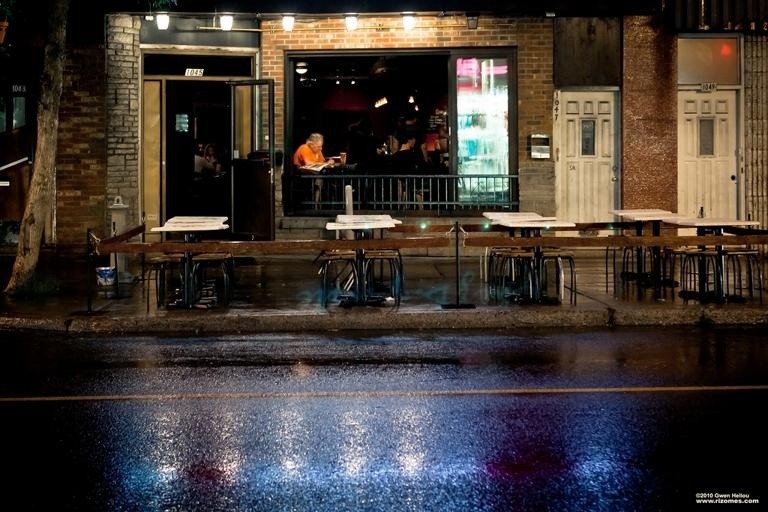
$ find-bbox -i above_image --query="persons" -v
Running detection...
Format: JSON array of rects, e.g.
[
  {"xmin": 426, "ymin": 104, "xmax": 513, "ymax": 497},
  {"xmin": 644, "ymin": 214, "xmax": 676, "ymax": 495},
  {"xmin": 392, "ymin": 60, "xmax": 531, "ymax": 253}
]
[
  {"xmin": 194, "ymin": 150, "xmax": 214, "ymax": 181},
  {"xmin": 203, "ymin": 143, "xmax": 224, "ymax": 175},
  {"xmin": 292, "ymin": 131, "xmax": 337, "ymax": 215},
  {"xmin": 196, "ymin": 142, "xmax": 205, "ymax": 157},
  {"xmin": 395, "ymin": 112, "xmax": 418, "ymax": 174}
]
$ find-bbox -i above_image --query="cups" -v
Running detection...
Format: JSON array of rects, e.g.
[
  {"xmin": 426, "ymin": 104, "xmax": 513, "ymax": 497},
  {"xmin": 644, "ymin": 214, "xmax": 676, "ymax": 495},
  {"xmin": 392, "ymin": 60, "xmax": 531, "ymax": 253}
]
[{"xmin": 340, "ymin": 152, "xmax": 347, "ymax": 168}]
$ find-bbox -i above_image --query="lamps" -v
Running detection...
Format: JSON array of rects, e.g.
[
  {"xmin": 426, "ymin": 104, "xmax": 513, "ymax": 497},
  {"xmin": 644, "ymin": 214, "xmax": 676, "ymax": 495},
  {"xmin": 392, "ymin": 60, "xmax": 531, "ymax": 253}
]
[{"xmin": 154, "ymin": 10, "xmax": 482, "ymax": 33}]
